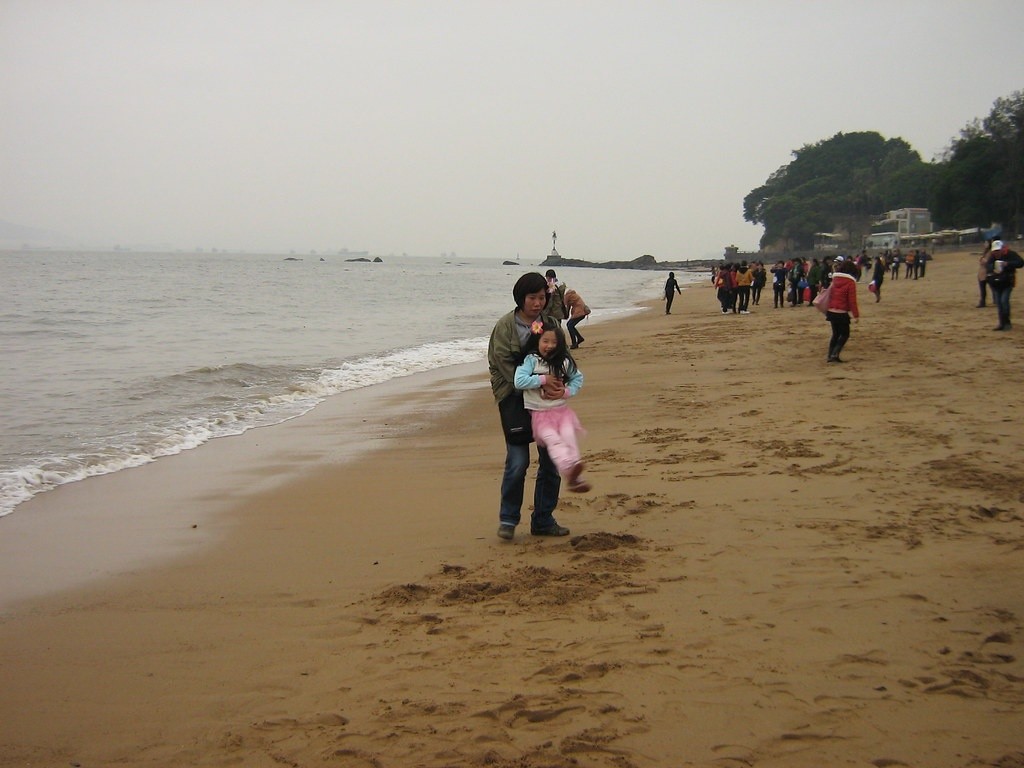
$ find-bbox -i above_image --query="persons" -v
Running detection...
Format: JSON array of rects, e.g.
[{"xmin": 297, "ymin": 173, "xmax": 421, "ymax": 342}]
[
  {"xmin": 563, "ymin": 285, "xmax": 586, "ymax": 349},
  {"xmin": 543, "ymin": 270, "xmax": 566, "ymax": 326},
  {"xmin": 664, "ymin": 272, "xmax": 681, "ymax": 315},
  {"xmin": 709, "ymin": 236, "xmax": 1001, "ymax": 363},
  {"xmin": 488, "ymin": 272, "xmax": 569, "ymax": 539},
  {"xmin": 514, "ymin": 320, "xmax": 591, "ymax": 493},
  {"xmin": 985, "ymin": 239, "xmax": 1024, "ymax": 331}
]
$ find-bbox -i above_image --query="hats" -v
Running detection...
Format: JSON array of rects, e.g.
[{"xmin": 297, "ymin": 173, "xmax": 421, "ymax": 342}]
[
  {"xmin": 991, "ymin": 240, "xmax": 1004, "ymax": 251},
  {"xmin": 545, "ymin": 269, "xmax": 556, "ymax": 278},
  {"xmin": 834, "ymin": 256, "xmax": 844, "ymax": 263}
]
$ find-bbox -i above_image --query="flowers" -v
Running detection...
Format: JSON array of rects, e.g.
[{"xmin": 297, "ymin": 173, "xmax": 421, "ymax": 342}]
[
  {"xmin": 529, "ymin": 320, "xmax": 544, "ymax": 336},
  {"xmin": 547, "ymin": 278, "xmax": 558, "ymax": 294}
]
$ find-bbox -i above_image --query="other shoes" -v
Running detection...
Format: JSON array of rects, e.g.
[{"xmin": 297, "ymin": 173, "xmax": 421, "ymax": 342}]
[
  {"xmin": 531, "ymin": 522, "xmax": 570, "ymax": 536},
  {"xmin": 993, "ymin": 324, "xmax": 1011, "ymax": 331},
  {"xmin": 568, "ymin": 484, "xmax": 591, "ymax": 493},
  {"xmin": 722, "ymin": 308, "xmax": 750, "ymax": 314},
  {"xmin": 497, "ymin": 523, "xmax": 515, "ymax": 539},
  {"xmin": 827, "ymin": 353, "xmax": 842, "ymax": 363},
  {"xmin": 568, "ymin": 461, "xmax": 583, "ymax": 482},
  {"xmin": 569, "ymin": 337, "xmax": 584, "ymax": 349}
]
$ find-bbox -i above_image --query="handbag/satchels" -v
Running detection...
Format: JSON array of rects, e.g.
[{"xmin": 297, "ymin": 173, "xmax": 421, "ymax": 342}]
[
  {"xmin": 584, "ymin": 305, "xmax": 591, "ymax": 315},
  {"xmin": 869, "ymin": 281, "xmax": 876, "ymax": 293},
  {"xmin": 562, "ymin": 304, "xmax": 568, "ymax": 319},
  {"xmin": 814, "ymin": 285, "xmax": 831, "ymax": 314},
  {"xmin": 498, "ymin": 392, "xmax": 535, "ymax": 444},
  {"xmin": 803, "ymin": 288, "xmax": 811, "ymax": 301},
  {"xmin": 787, "ymin": 290, "xmax": 793, "ymax": 303}
]
